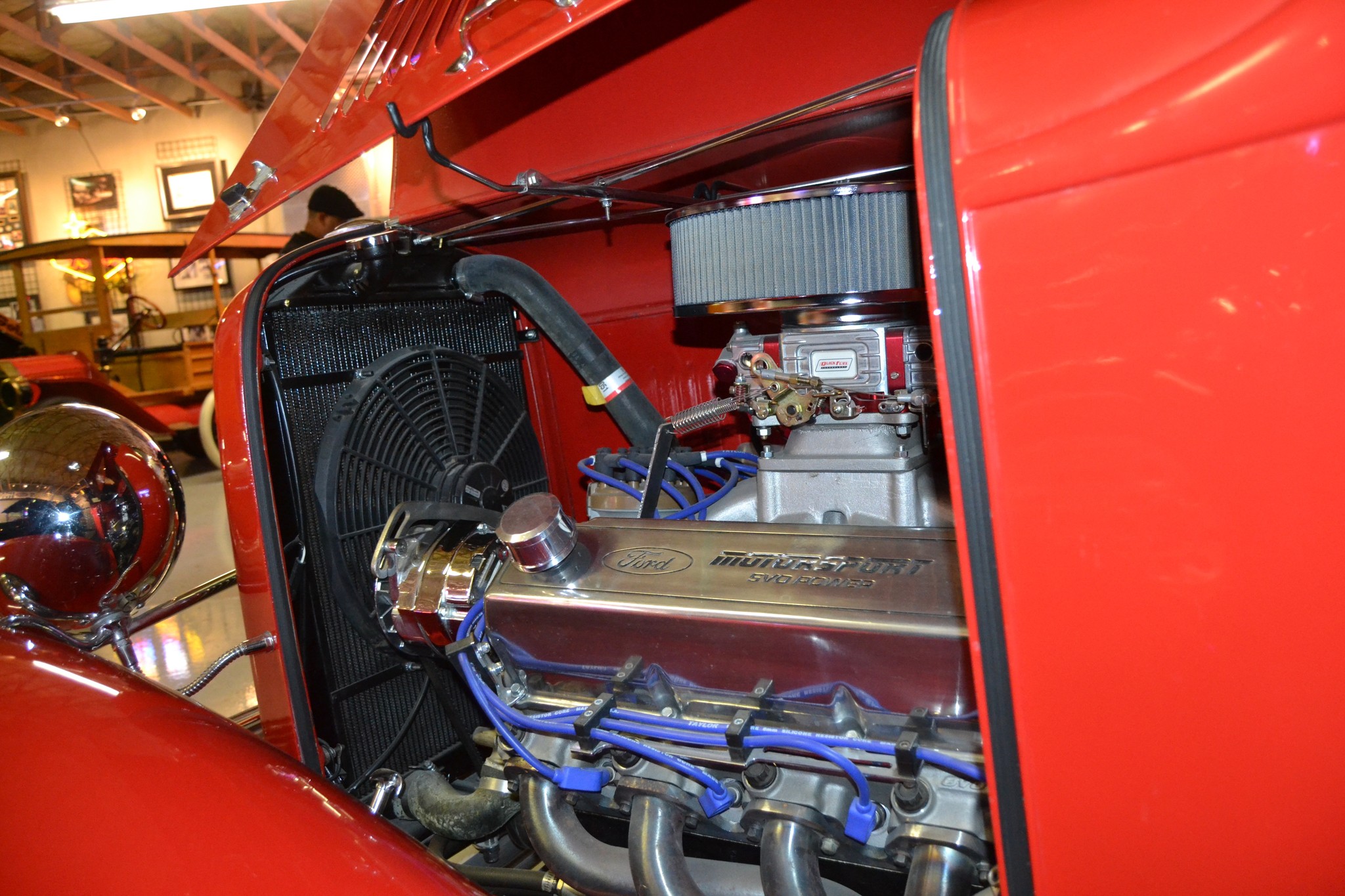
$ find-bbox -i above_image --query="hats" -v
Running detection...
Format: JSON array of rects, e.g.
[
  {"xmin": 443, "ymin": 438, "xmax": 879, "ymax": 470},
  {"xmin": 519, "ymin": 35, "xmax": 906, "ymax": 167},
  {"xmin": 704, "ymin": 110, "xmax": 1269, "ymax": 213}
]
[{"xmin": 308, "ymin": 184, "xmax": 365, "ymax": 219}]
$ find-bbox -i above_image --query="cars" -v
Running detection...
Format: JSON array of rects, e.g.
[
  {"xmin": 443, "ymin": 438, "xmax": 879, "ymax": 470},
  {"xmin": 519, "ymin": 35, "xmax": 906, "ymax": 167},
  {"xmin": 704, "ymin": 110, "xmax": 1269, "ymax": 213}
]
[{"xmin": 0, "ymin": 230, "xmax": 327, "ymax": 467}]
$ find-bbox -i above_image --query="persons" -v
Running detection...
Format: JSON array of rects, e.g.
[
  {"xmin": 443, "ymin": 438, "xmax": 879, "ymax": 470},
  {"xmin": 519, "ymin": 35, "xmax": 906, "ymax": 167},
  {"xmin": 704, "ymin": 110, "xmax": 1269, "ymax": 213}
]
[{"xmin": 272, "ymin": 184, "xmax": 366, "ymax": 262}]
